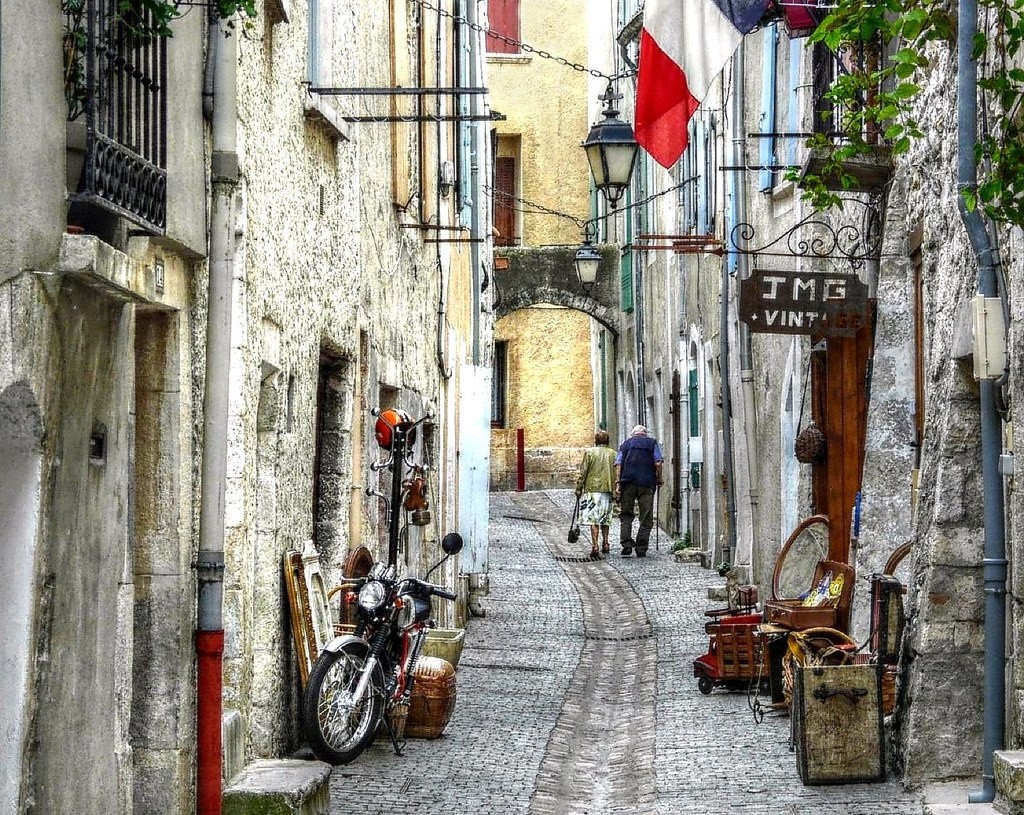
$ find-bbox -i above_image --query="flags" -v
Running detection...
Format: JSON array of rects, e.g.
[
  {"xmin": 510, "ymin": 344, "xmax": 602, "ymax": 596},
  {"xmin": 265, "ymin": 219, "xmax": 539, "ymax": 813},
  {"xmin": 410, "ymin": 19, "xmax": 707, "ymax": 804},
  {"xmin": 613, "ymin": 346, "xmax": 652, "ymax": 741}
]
[{"xmin": 634, "ymin": 1, "xmax": 770, "ymax": 170}]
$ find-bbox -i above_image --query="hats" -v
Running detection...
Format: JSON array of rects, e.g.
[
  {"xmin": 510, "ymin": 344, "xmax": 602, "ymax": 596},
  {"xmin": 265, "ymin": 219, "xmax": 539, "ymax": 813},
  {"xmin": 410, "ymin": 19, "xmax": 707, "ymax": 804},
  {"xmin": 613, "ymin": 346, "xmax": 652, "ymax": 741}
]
[{"xmin": 630, "ymin": 425, "xmax": 647, "ymax": 434}]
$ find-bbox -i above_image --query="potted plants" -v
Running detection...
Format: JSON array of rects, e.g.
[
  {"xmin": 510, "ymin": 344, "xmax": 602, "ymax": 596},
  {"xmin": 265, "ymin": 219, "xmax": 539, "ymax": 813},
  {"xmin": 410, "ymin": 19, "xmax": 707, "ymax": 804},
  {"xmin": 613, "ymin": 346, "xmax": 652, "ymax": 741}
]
[{"xmin": 494, "ymin": 246, "xmax": 511, "ymax": 269}]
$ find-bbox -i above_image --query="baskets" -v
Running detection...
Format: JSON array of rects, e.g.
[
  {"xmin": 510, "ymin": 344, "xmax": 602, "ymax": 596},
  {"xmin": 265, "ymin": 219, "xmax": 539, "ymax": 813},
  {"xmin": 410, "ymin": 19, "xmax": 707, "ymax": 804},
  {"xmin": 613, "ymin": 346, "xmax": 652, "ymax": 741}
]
[
  {"xmin": 399, "ymin": 655, "xmax": 456, "ymax": 739},
  {"xmin": 783, "ymin": 626, "xmax": 898, "ymax": 725}
]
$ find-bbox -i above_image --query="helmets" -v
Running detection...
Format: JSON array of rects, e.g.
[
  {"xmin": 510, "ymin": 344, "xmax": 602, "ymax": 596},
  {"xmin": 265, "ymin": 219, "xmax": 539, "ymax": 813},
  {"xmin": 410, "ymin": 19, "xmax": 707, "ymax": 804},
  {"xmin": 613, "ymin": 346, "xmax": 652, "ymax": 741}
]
[{"xmin": 375, "ymin": 408, "xmax": 416, "ymax": 448}]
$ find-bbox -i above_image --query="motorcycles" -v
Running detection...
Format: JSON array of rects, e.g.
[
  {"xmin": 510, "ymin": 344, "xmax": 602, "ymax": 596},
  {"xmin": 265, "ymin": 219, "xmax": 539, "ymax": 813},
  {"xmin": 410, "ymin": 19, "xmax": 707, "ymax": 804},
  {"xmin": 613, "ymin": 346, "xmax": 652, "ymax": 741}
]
[{"xmin": 304, "ymin": 533, "xmax": 464, "ymax": 767}]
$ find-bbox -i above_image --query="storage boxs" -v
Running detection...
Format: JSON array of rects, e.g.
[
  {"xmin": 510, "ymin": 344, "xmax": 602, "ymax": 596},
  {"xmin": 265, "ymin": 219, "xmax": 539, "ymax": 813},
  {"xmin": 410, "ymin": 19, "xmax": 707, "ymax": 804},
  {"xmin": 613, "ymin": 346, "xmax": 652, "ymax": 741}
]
[
  {"xmin": 764, "ymin": 560, "xmax": 855, "ymax": 628},
  {"xmin": 402, "ymin": 654, "xmax": 456, "ymax": 738},
  {"xmin": 793, "ymin": 574, "xmax": 905, "ymax": 787}
]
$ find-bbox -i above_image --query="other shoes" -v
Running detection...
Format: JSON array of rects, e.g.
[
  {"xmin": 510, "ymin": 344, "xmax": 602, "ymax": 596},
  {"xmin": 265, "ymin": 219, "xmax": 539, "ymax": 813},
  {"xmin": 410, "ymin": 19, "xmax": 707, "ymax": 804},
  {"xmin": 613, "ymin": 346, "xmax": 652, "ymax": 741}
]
[
  {"xmin": 621, "ymin": 547, "xmax": 632, "ymax": 555},
  {"xmin": 638, "ymin": 552, "xmax": 645, "ymax": 557}
]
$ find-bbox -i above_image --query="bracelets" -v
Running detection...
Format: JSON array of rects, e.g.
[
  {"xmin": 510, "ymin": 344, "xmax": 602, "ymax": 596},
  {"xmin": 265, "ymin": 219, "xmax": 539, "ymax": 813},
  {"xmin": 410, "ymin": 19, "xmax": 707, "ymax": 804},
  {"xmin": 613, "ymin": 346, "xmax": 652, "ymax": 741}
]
[{"xmin": 614, "ymin": 480, "xmax": 620, "ymax": 483}]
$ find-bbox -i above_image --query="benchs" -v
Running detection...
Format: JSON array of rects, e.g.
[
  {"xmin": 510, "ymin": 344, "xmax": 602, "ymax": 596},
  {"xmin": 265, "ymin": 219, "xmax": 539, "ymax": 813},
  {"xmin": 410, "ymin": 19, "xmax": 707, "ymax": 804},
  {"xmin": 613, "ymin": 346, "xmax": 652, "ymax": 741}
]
[{"xmin": 222, "ymin": 760, "xmax": 331, "ymax": 815}]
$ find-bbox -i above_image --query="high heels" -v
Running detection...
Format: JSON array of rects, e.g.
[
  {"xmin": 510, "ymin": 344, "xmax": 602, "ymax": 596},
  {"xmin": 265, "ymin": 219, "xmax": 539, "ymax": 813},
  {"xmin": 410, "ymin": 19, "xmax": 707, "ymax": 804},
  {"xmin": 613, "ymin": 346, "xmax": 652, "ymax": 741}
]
[
  {"xmin": 590, "ymin": 547, "xmax": 599, "ymax": 556},
  {"xmin": 602, "ymin": 543, "xmax": 610, "ymax": 553}
]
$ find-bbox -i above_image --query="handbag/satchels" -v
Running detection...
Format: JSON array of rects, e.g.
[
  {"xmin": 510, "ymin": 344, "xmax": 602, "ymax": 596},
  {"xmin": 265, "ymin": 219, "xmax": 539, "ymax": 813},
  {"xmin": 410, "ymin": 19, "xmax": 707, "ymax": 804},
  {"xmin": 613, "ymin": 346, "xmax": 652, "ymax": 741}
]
[
  {"xmin": 568, "ymin": 497, "xmax": 581, "ymax": 543},
  {"xmin": 795, "ymin": 427, "xmax": 825, "ymax": 463}
]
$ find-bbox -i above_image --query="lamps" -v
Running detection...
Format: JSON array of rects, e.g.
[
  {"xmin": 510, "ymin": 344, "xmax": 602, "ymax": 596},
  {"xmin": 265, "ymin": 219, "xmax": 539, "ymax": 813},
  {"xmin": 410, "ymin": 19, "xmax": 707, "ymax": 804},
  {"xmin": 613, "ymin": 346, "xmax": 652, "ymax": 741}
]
[
  {"xmin": 571, "ymin": 223, "xmax": 603, "ymax": 296},
  {"xmin": 579, "ymin": 83, "xmax": 639, "ymax": 209}
]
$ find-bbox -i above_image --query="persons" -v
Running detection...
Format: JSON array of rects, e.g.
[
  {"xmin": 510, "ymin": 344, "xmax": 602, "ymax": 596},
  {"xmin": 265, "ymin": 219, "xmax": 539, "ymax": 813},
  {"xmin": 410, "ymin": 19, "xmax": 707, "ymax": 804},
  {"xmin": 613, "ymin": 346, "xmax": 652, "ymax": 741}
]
[
  {"xmin": 575, "ymin": 430, "xmax": 617, "ymax": 557},
  {"xmin": 613, "ymin": 424, "xmax": 663, "ymax": 558}
]
